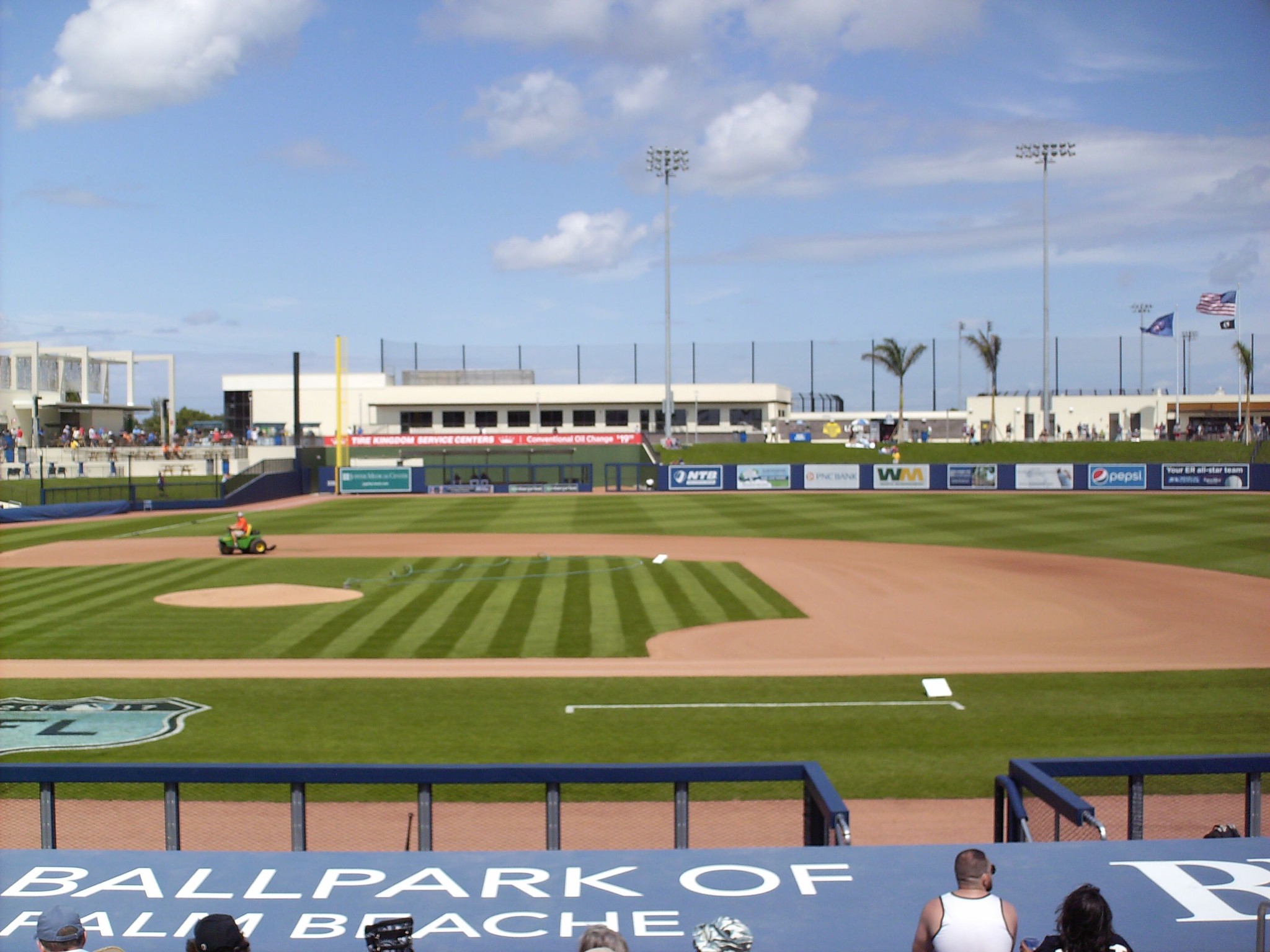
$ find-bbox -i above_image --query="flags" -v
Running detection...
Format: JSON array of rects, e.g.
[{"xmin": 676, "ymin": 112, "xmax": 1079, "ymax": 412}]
[
  {"xmin": 1197, "ymin": 290, "xmax": 1238, "ymax": 329},
  {"xmin": 1140, "ymin": 311, "xmax": 1176, "ymax": 337}
]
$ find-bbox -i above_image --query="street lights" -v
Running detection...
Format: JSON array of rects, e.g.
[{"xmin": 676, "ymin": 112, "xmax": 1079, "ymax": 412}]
[
  {"xmin": 645, "ymin": 147, "xmax": 691, "ymax": 446},
  {"xmin": 1016, "ymin": 140, "xmax": 1075, "ymax": 441}
]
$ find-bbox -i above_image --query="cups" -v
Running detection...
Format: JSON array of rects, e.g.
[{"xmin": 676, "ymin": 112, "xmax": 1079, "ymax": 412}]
[{"xmin": 1024, "ymin": 937, "xmax": 1040, "ymax": 951}]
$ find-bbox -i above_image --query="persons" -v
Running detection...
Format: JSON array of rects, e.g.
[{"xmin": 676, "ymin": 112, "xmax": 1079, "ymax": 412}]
[
  {"xmin": 913, "ymin": 848, "xmax": 1018, "ymax": 952},
  {"xmin": 1019, "ymin": 884, "xmax": 1133, "ymax": 952},
  {"xmin": 762, "ymin": 422, "xmax": 777, "ymax": 443},
  {"xmin": 668, "ymin": 456, "xmax": 685, "ymax": 465},
  {"xmin": 581, "ymin": 925, "xmax": 629, "ymax": 952},
  {"xmin": 692, "ymin": 916, "xmax": 754, "ymax": 952},
  {"xmin": 0, "ymin": 424, "xmax": 282, "ymax": 462},
  {"xmin": 229, "ymin": 512, "xmax": 247, "ymax": 554},
  {"xmin": 347, "ymin": 424, "xmax": 363, "ymax": 436},
  {"xmin": 892, "ymin": 446, "xmax": 900, "ymax": 464},
  {"xmin": 186, "ymin": 914, "xmax": 249, "ymax": 952},
  {"xmin": 666, "ymin": 436, "xmax": 681, "ymax": 450},
  {"xmin": 33, "ymin": 905, "xmax": 88, "ymax": 952},
  {"xmin": 920, "ymin": 419, "xmax": 1268, "ymax": 442},
  {"xmin": 450, "ymin": 465, "xmax": 490, "ymax": 485}
]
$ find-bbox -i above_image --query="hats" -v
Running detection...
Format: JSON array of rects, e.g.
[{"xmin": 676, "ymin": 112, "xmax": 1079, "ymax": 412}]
[
  {"xmin": 33, "ymin": 905, "xmax": 84, "ymax": 942},
  {"xmin": 194, "ymin": 914, "xmax": 244, "ymax": 952},
  {"xmin": 365, "ymin": 918, "xmax": 413, "ymax": 952},
  {"xmin": 692, "ymin": 915, "xmax": 754, "ymax": 952}
]
